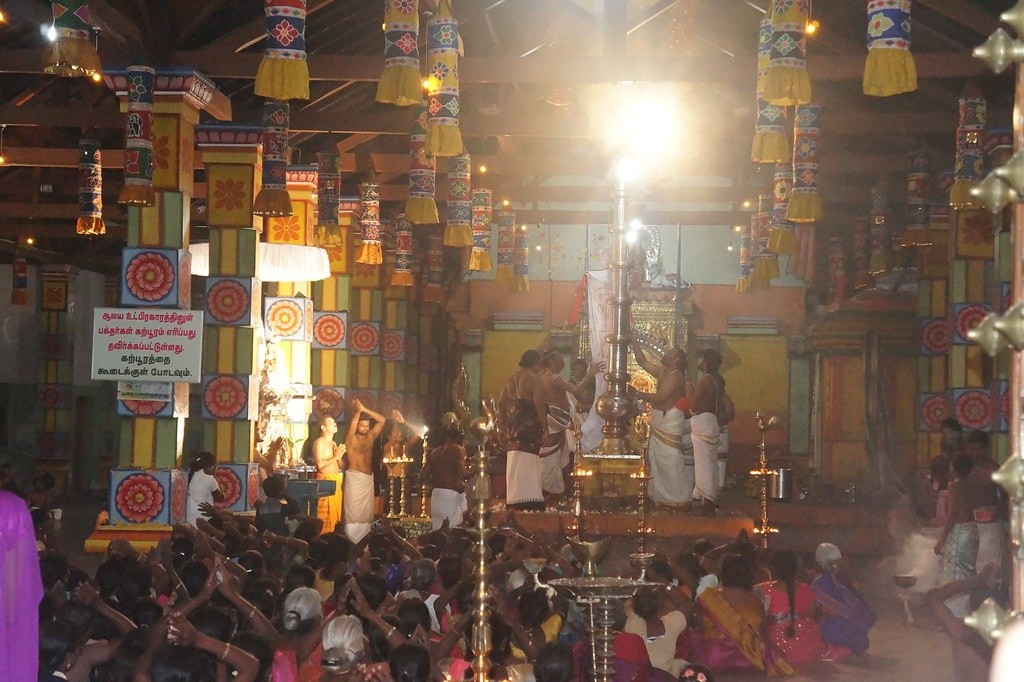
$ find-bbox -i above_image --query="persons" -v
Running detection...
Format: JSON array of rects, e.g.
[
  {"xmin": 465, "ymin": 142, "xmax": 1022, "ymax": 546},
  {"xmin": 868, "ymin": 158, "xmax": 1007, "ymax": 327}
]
[{"xmin": 0, "ymin": 345, "xmax": 1010, "ymax": 682}]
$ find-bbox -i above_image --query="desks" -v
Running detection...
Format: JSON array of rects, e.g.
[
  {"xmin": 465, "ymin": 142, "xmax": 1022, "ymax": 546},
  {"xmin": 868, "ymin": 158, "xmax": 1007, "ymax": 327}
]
[
  {"xmin": 287, "ymin": 479, "xmax": 337, "ymax": 517},
  {"xmin": 486, "ymin": 507, "xmax": 755, "ymax": 577}
]
